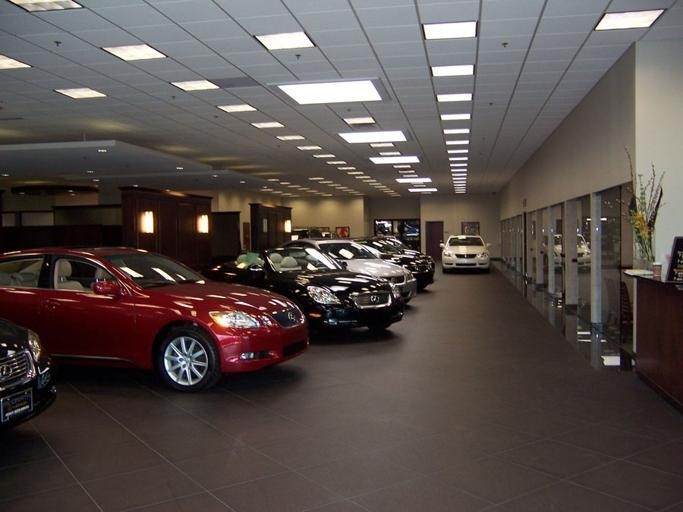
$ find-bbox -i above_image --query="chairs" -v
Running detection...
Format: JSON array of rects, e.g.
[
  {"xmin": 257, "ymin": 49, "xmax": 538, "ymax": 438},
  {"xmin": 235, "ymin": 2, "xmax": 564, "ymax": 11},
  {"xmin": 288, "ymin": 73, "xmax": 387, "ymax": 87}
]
[
  {"xmin": 279, "ymin": 256, "xmax": 302, "ymax": 273},
  {"xmin": 90, "ymin": 267, "xmax": 113, "ymax": 291},
  {"xmin": 57, "ymin": 259, "xmax": 84, "ymax": 290},
  {"xmin": 269, "ymin": 253, "xmax": 283, "ymax": 270}
]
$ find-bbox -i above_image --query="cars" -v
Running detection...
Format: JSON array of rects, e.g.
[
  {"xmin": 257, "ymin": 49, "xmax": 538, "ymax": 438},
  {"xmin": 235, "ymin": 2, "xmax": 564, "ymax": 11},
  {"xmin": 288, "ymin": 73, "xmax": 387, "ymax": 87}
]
[
  {"xmin": 201, "ymin": 233, "xmax": 435, "ymax": 339},
  {"xmin": 542, "ymin": 233, "xmax": 591, "ymax": 270},
  {"xmin": 377, "ymin": 224, "xmax": 420, "ymax": 237},
  {"xmin": 439, "ymin": 234, "xmax": 492, "ymax": 271},
  {"xmin": 0, "ymin": 319, "xmax": 57, "ymax": 432},
  {"xmin": 1, "ymin": 246, "xmax": 308, "ymax": 393}
]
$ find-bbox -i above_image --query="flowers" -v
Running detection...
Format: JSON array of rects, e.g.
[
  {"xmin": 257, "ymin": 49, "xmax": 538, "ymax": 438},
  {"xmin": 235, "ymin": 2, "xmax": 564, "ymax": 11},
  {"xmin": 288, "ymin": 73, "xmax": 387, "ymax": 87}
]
[{"xmin": 613, "ymin": 144, "xmax": 665, "ymax": 264}]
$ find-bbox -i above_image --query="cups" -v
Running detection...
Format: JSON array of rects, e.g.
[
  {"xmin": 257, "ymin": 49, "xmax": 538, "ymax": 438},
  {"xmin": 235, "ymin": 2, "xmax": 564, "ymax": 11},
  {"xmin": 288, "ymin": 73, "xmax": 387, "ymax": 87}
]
[{"xmin": 653, "ymin": 262, "xmax": 663, "ymax": 277}]
